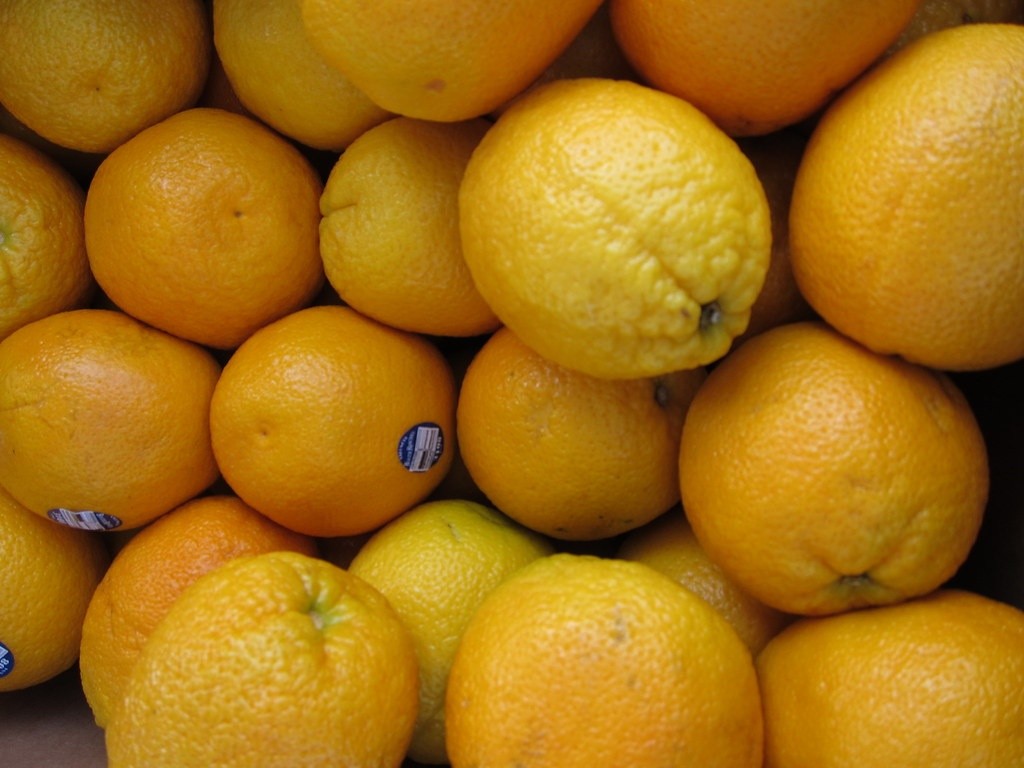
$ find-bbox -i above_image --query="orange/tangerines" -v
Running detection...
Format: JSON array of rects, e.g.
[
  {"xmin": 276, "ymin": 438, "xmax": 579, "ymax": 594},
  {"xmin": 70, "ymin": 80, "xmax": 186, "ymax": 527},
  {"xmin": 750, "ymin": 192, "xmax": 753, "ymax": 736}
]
[{"xmin": 0, "ymin": 0, "xmax": 1024, "ymax": 768}]
